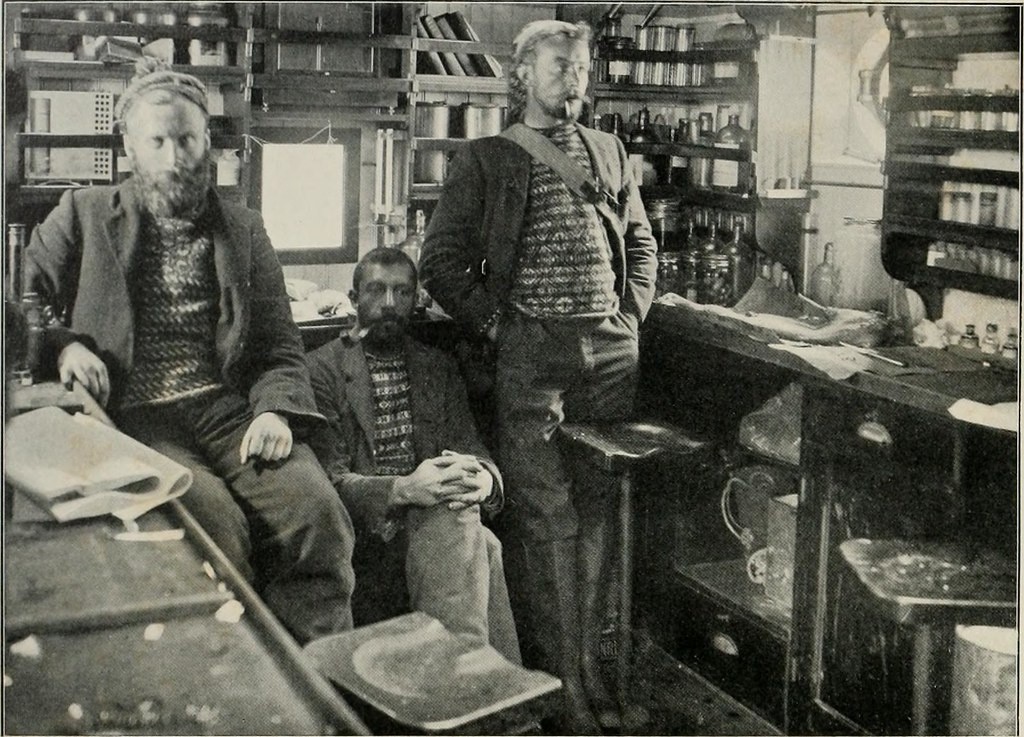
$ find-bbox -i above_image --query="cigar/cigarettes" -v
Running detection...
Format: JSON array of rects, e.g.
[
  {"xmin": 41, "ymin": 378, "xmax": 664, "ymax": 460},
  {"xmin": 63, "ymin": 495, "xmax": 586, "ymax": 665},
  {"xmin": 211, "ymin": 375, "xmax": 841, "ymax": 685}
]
[{"xmin": 565, "ymin": 98, "xmax": 572, "ymax": 119}]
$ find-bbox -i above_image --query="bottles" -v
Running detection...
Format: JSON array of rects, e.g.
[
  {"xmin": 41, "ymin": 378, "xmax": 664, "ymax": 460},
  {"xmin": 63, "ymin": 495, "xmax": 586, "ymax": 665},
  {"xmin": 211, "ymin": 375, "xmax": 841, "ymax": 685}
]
[
  {"xmin": 604, "ymin": 18, "xmax": 621, "ymax": 48},
  {"xmin": 961, "ymin": 325, "xmax": 979, "ymax": 349},
  {"xmin": 610, "ymin": 113, "xmax": 627, "ymax": 150},
  {"xmin": 630, "ymin": 109, "xmax": 656, "ymax": 188},
  {"xmin": 400, "ymin": 210, "xmax": 427, "ymax": 263},
  {"xmin": 656, "ymin": 218, "xmax": 671, "ymax": 252},
  {"xmin": 1002, "ymin": 334, "xmax": 1017, "ymax": 359},
  {"xmin": 809, "ymin": 242, "xmax": 841, "ymax": 322},
  {"xmin": 671, "ymin": 118, "xmax": 691, "ymax": 186},
  {"xmin": 711, "ymin": 116, "xmax": 751, "ymax": 195},
  {"xmin": 981, "ymin": 324, "xmax": 998, "ymax": 355},
  {"xmin": 692, "ymin": 113, "xmax": 716, "ymax": 190},
  {"xmin": 700, "ymin": 220, "xmax": 724, "ymax": 254},
  {"xmin": 720, "ymin": 223, "xmax": 751, "ymax": 255},
  {"xmin": 6, "ymin": 224, "xmax": 43, "ymax": 374},
  {"xmin": 680, "ymin": 219, "xmax": 701, "ymax": 251}
]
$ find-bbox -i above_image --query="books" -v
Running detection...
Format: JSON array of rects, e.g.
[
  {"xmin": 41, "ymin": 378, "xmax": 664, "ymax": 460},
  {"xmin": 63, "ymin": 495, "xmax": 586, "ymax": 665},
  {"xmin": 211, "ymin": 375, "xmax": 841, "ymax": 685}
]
[{"xmin": 421, "ymin": 11, "xmax": 501, "ymax": 77}]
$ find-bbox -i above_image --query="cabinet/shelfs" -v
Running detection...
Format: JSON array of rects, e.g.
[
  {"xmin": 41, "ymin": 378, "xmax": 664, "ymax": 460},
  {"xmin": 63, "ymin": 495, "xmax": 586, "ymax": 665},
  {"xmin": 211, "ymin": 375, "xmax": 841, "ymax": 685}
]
[
  {"xmin": 1, "ymin": 0, "xmax": 512, "ymax": 735},
  {"xmin": 586, "ymin": 4, "xmax": 1020, "ymax": 737}
]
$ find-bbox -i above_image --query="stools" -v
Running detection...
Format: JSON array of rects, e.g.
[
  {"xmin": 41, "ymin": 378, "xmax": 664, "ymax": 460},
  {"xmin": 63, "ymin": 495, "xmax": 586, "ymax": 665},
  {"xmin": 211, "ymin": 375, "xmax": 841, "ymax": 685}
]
[
  {"xmin": 300, "ymin": 612, "xmax": 563, "ymax": 736},
  {"xmin": 558, "ymin": 413, "xmax": 713, "ymax": 737}
]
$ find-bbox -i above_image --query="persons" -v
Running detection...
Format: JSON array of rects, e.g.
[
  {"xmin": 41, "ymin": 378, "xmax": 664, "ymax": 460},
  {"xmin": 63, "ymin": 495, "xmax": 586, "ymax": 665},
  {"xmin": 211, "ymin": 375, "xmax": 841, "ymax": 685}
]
[
  {"xmin": 420, "ymin": 20, "xmax": 659, "ymax": 735},
  {"xmin": 4, "ymin": 71, "xmax": 357, "ymax": 642},
  {"xmin": 296, "ymin": 246, "xmax": 549, "ymax": 735}
]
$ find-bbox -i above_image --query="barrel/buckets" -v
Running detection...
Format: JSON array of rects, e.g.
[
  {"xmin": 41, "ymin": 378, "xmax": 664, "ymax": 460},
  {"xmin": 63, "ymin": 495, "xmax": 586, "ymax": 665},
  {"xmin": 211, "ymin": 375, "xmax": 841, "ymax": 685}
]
[{"xmin": 949, "ymin": 624, "xmax": 1016, "ymax": 736}]
